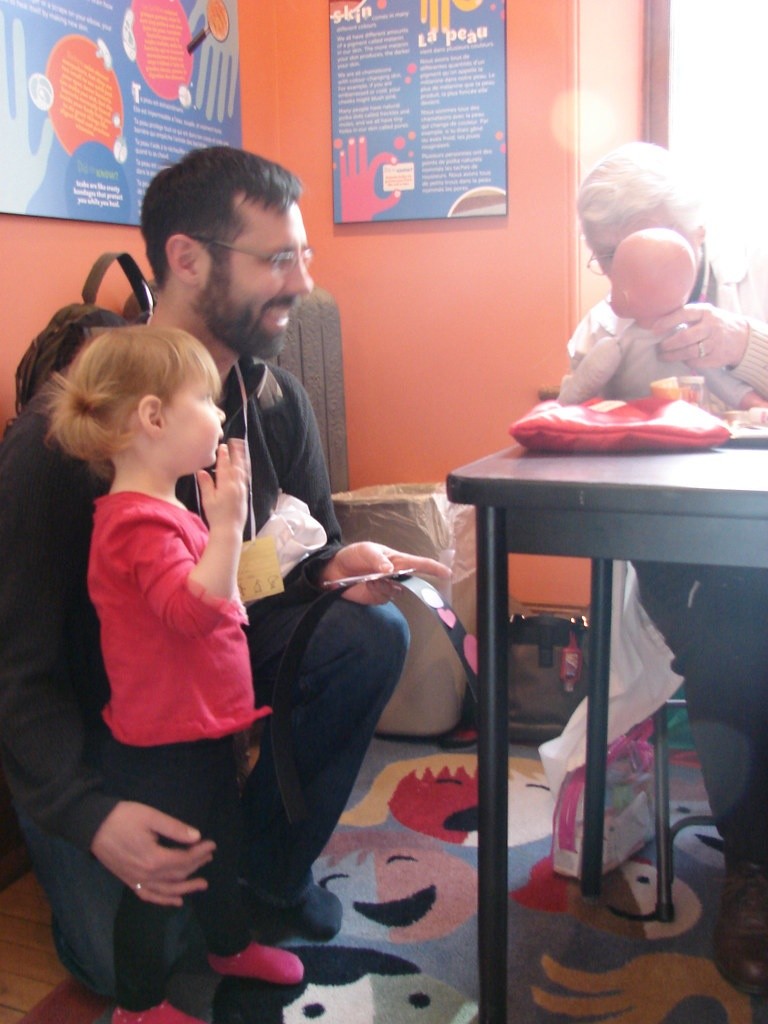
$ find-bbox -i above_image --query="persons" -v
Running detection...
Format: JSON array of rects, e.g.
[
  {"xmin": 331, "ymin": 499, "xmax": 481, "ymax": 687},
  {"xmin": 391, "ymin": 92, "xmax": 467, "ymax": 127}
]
[
  {"xmin": 48, "ymin": 325, "xmax": 305, "ymax": 1024},
  {"xmin": 557, "ymin": 227, "xmax": 768, "ymax": 409},
  {"xmin": 0, "ymin": 146, "xmax": 451, "ymax": 1024},
  {"xmin": 535, "ymin": 143, "xmax": 768, "ymax": 997}
]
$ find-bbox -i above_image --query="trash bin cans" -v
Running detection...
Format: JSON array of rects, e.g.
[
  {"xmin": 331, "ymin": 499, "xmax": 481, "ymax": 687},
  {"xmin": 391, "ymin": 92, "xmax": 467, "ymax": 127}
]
[{"xmin": 333, "ymin": 481, "xmax": 478, "ymax": 737}]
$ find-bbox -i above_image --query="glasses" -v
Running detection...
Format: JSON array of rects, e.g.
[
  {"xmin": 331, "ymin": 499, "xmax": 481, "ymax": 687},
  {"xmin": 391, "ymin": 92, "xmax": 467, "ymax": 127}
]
[
  {"xmin": 585, "ymin": 246, "xmax": 617, "ymax": 274},
  {"xmin": 205, "ymin": 238, "xmax": 312, "ymax": 271}
]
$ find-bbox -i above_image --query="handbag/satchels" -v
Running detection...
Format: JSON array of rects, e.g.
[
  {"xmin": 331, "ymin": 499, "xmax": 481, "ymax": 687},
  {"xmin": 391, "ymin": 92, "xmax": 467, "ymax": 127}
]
[{"xmin": 548, "ymin": 718, "xmax": 659, "ymax": 882}]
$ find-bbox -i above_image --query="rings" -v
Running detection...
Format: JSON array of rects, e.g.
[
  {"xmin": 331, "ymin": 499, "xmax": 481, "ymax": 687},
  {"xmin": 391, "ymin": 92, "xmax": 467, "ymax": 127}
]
[
  {"xmin": 698, "ymin": 342, "xmax": 705, "ymax": 357},
  {"xmin": 136, "ymin": 883, "xmax": 143, "ymax": 889}
]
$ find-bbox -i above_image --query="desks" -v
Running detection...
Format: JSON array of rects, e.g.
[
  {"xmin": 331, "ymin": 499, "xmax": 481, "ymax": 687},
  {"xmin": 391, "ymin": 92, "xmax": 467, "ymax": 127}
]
[{"xmin": 447, "ymin": 435, "xmax": 766, "ymax": 1023}]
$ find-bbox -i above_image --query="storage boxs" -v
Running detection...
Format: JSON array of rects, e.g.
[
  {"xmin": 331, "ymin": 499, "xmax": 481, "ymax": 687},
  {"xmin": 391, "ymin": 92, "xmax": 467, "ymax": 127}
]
[{"xmin": 506, "ymin": 601, "xmax": 589, "ymax": 736}]
